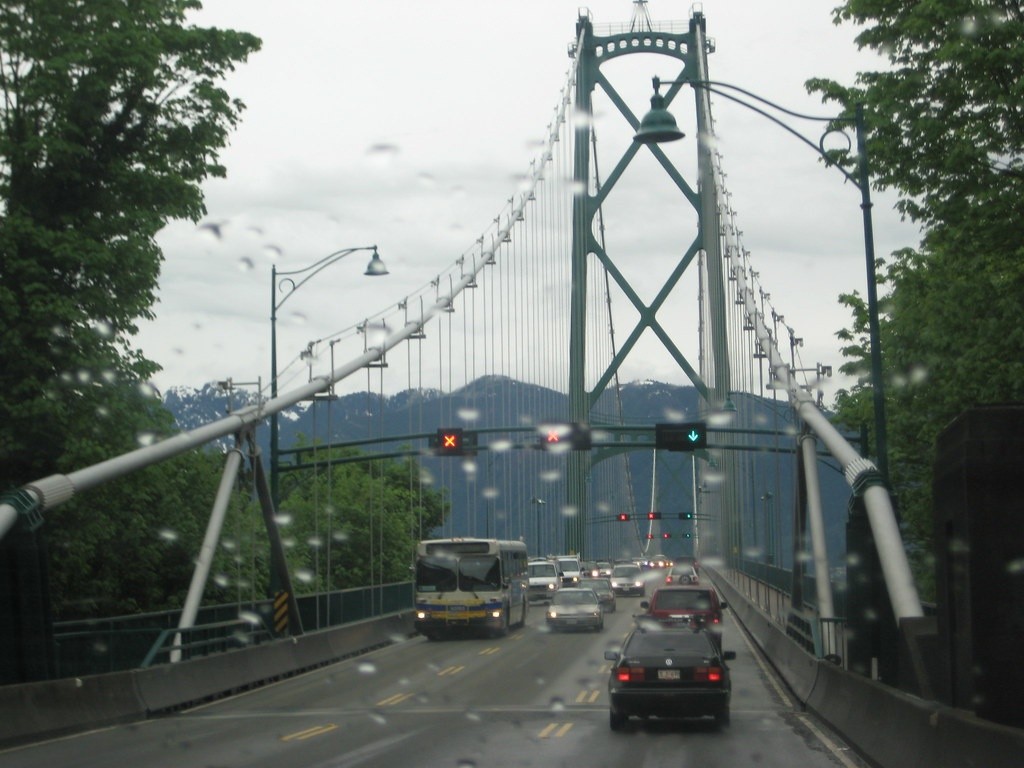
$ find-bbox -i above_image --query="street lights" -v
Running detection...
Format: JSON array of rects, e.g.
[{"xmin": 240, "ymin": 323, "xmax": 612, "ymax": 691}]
[
  {"xmin": 268, "ymin": 245, "xmax": 389, "ymax": 597},
  {"xmin": 530, "ymin": 496, "xmax": 546, "ymax": 558},
  {"xmin": 761, "ymin": 492, "xmax": 774, "ymax": 563},
  {"xmin": 633, "ymin": 75, "xmax": 900, "ymax": 689}
]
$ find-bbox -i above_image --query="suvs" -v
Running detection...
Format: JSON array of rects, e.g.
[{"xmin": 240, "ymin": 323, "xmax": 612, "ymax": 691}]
[
  {"xmin": 641, "ymin": 585, "xmax": 728, "ymax": 648},
  {"xmin": 667, "ymin": 556, "xmax": 700, "ymax": 586},
  {"xmin": 527, "ymin": 553, "xmax": 581, "ymax": 600}
]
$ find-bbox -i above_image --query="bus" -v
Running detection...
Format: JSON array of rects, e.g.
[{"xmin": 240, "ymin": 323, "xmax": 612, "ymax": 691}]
[{"xmin": 413, "ymin": 538, "xmax": 530, "ymax": 639}]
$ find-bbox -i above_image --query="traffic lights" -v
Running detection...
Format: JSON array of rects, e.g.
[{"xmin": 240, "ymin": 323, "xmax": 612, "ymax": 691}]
[
  {"xmin": 648, "ymin": 512, "xmax": 662, "ymax": 520},
  {"xmin": 685, "ymin": 532, "xmax": 690, "ymax": 538},
  {"xmin": 664, "ymin": 533, "xmax": 670, "ymax": 539},
  {"xmin": 618, "ymin": 514, "xmax": 630, "ymax": 521},
  {"xmin": 679, "ymin": 512, "xmax": 692, "ymax": 520},
  {"xmin": 540, "ymin": 422, "xmax": 592, "ymax": 451},
  {"xmin": 437, "ymin": 428, "xmax": 462, "ymax": 456},
  {"xmin": 656, "ymin": 423, "xmax": 707, "ymax": 450},
  {"xmin": 648, "ymin": 534, "xmax": 653, "ymax": 539}
]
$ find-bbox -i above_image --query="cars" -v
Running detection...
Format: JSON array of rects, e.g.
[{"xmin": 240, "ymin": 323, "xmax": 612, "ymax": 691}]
[
  {"xmin": 605, "ymin": 628, "xmax": 737, "ymax": 731},
  {"xmin": 543, "ymin": 588, "xmax": 604, "ymax": 631},
  {"xmin": 577, "ymin": 578, "xmax": 616, "ymax": 613},
  {"xmin": 581, "ymin": 555, "xmax": 675, "ymax": 597}
]
